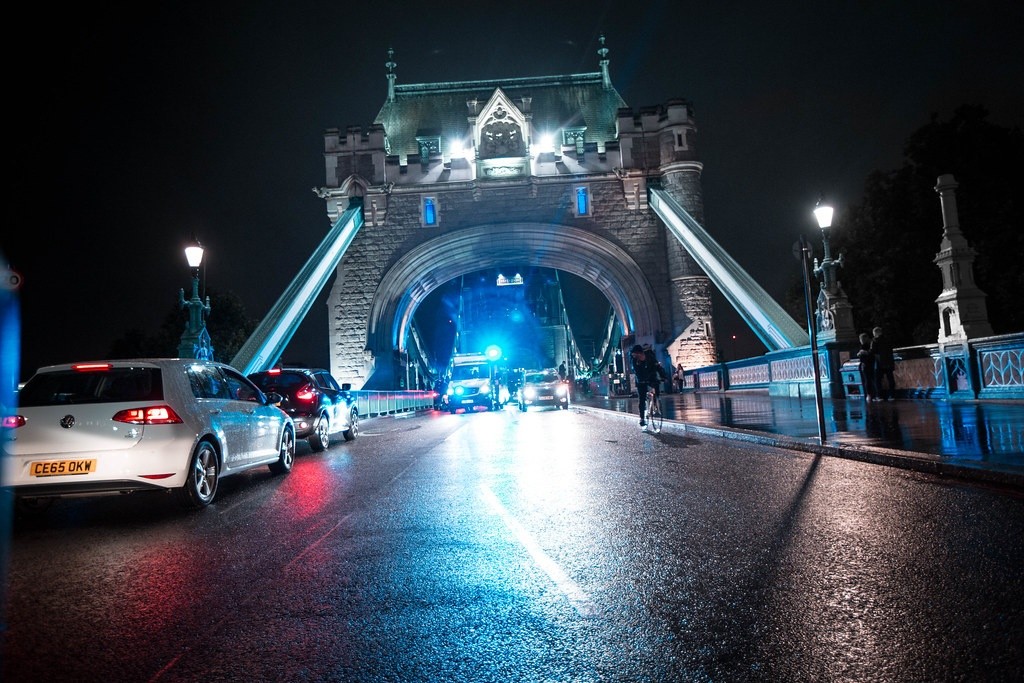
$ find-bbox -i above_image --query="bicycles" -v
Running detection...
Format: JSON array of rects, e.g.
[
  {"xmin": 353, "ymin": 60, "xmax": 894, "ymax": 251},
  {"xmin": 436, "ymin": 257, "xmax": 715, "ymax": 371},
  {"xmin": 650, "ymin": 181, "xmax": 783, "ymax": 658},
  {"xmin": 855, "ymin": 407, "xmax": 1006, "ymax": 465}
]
[{"xmin": 635, "ymin": 378, "xmax": 667, "ymax": 433}]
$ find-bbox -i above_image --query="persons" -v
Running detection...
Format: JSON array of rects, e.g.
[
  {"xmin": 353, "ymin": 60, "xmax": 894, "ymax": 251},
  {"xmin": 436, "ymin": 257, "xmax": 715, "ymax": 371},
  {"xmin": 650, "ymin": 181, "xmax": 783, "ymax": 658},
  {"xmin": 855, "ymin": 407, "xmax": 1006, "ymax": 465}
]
[
  {"xmin": 558, "ymin": 361, "xmax": 566, "ymax": 380},
  {"xmin": 579, "ymin": 371, "xmax": 625, "ymax": 396},
  {"xmin": 631, "ymin": 343, "xmax": 665, "ymax": 426},
  {"xmin": 675, "ymin": 364, "xmax": 684, "ymax": 395},
  {"xmin": 857, "ymin": 327, "xmax": 897, "ymax": 402}
]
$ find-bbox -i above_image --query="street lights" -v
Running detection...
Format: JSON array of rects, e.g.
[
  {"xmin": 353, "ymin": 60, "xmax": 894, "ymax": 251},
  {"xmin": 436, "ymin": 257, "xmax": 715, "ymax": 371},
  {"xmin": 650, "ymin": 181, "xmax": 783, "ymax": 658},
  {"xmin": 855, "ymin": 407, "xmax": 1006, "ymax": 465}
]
[
  {"xmin": 173, "ymin": 234, "xmax": 219, "ymax": 361},
  {"xmin": 808, "ymin": 193, "xmax": 858, "ymax": 346}
]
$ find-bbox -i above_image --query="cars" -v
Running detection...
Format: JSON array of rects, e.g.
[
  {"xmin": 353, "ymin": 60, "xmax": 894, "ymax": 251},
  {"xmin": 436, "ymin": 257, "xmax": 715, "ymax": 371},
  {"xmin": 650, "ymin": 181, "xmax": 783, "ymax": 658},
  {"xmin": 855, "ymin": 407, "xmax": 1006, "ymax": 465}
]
[
  {"xmin": 514, "ymin": 370, "xmax": 570, "ymax": 411},
  {"xmin": 0, "ymin": 356, "xmax": 299, "ymax": 509},
  {"xmin": 235, "ymin": 366, "xmax": 360, "ymax": 451}
]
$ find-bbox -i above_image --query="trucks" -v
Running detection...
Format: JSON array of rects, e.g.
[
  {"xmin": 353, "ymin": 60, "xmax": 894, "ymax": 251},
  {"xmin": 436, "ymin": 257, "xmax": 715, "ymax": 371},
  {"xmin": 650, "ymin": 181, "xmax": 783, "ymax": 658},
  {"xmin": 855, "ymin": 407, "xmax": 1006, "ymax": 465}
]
[{"xmin": 445, "ymin": 353, "xmax": 508, "ymax": 415}]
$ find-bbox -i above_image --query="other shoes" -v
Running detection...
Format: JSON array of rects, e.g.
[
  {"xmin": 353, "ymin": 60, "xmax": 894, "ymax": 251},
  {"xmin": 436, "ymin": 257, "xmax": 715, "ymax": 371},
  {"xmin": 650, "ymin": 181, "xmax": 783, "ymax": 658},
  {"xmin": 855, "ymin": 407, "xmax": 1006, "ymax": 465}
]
[
  {"xmin": 872, "ymin": 397, "xmax": 884, "ymax": 401},
  {"xmin": 888, "ymin": 396, "xmax": 896, "ymax": 401},
  {"xmin": 640, "ymin": 419, "xmax": 644, "ymax": 426}
]
